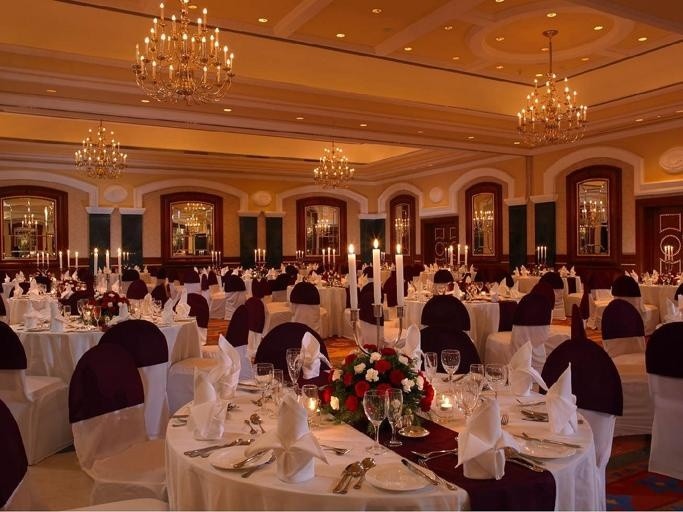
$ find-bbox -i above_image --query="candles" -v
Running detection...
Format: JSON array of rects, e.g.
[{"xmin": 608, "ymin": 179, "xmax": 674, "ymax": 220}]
[
  {"xmin": 37, "ymin": 248, "xmax": 122, "ymax": 278},
  {"xmin": 444, "ymin": 244, "xmax": 468, "ymax": 266},
  {"xmin": 255, "ymin": 249, "xmax": 265, "ymax": 262},
  {"xmin": 664, "ymin": 246, "xmax": 673, "ymax": 262},
  {"xmin": 346, "ymin": 240, "xmax": 404, "ymax": 308},
  {"xmin": 296, "ymin": 250, "xmax": 304, "ymax": 260},
  {"xmin": 536, "ymin": 246, "xmax": 547, "ymax": 264},
  {"xmin": 211, "ymin": 251, "xmax": 221, "ymax": 262},
  {"xmin": 322, "ymin": 247, "xmax": 336, "ymax": 265}
]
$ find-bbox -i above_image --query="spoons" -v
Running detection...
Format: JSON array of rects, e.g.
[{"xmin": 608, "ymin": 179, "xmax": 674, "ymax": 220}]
[
  {"xmin": 250, "ymin": 414, "xmax": 266, "ymax": 433},
  {"xmin": 332, "ymin": 458, "xmax": 375, "ymax": 494}
]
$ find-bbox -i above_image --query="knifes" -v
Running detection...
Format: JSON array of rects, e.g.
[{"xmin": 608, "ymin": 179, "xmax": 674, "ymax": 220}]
[
  {"xmin": 233, "ymin": 447, "xmax": 273, "ymax": 469},
  {"xmin": 401, "ymin": 459, "xmax": 440, "ymax": 486},
  {"xmin": 513, "ymin": 434, "xmax": 580, "ymax": 448}
]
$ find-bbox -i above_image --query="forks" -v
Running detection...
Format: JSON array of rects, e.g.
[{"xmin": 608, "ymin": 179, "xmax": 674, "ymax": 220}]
[
  {"xmin": 244, "ymin": 420, "xmax": 257, "ymax": 434},
  {"xmin": 320, "ymin": 444, "xmax": 353, "ymax": 456},
  {"xmin": 410, "ymin": 447, "xmax": 458, "ymax": 459}
]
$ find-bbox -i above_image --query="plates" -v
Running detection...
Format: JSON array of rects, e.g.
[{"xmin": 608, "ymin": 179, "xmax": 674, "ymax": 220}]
[
  {"xmin": 236, "ymin": 380, "xmax": 264, "ymax": 391},
  {"xmin": 28, "ymin": 315, "xmax": 196, "ymax": 332},
  {"xmin": 364, "ymin": 461, "xmax": 435, "ymax": 492},
  {"xmin": 208, "ymin": 446, "xmax": 273, "ymax": 469},
  {"xmin": 518, "ymin": 438, "xmax": 576, "ymax": 458},
  {"xmin": 178, "ymin": 413, "xmax": 188, "ymax": 422}
]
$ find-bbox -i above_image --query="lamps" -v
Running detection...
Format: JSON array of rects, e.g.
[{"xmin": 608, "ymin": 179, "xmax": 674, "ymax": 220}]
[
  {"xmin": 516, "ymin": 30, "xmax": 590, "ymax": 148},
  {"xmin": 395, "ymin": 218, "xmax": 409, "ymax": 231},
  {"xmin": 582, "ymin": 200, "xmax": 606, "ymax": 228},
  {"xmin": 182, "ymin": 202, "xmax": 206, "ymax": 238},
  {"xmin": 130, "ymin": 0, "xmax": 236, "ymax": 107},
  {"xmin": 73, "ymin": 121, "xmax": 129, "ymax": 180},
  {"xmin": 315, "ymin": 219, "xmax": 330, "ymax": 235},
  {"xmin": 474, "ymin": 209, "xmax": 493, "ymax": 233},
  {"xmin": 21, "ymin": 208, "xmax": 38, "ymax": 229},
  {"xmin": 313, "ymin": 141, "xmax": 354, "ymax": 192}
]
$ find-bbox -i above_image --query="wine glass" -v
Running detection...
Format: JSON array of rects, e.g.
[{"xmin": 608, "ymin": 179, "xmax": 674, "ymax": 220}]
[
  {"xmin": 640, "ymin": 272, "xmax": 681, "ymax": 286},
  {"xmin": 64, "ymin": 298, "xmax": 162, "ymax": 329},
  {"xmin": 409, "ymin": 279, "xmax": 510, "ymax": 298},
  {"xmin": 328, "ymin": 276, "xmax": 333, "ymax": 287},
  {"xmin": 253, "ymin": 347, "xmax": 507, "ymax": 454}
]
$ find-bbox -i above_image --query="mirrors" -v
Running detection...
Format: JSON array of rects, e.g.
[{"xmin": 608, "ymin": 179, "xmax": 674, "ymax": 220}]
[
  {"xmin": 465, "ymin": 182, "xmax": 502, "ymax": 265},
  {"xmin": 565, "ymin": 165, "xmax": 622, "ymax": 261},
  {"xmin": 0, "ymin": 184, "xmax": 69, "ymax": 259},
  {"xmin": 160, "ymin": 191, "xmax": 224, "ymax": 262},
  {"xmin": 295, "ymin": 196, "xmax": 348, "ymax": 261},
  {"xmin": 390, "ymin": 194, "xmax": 416, "ymax": 256}
]
